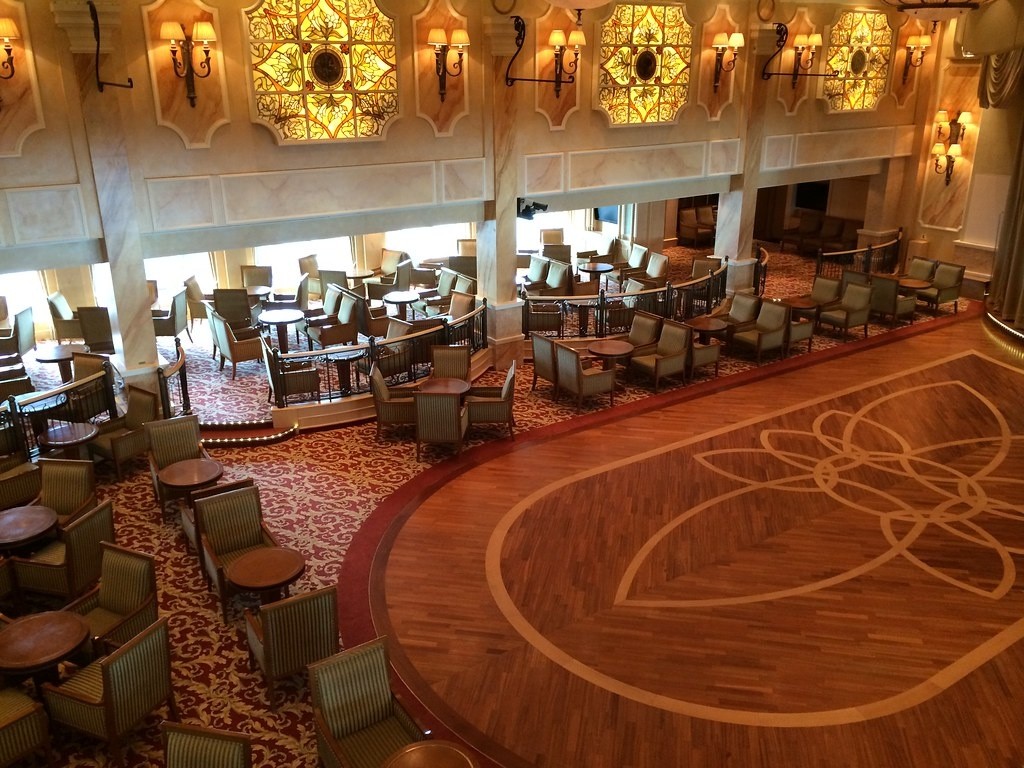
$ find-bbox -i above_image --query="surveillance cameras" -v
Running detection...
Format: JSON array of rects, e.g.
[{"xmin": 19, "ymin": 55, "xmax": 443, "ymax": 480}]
[
  {"xmin": 534, "ymin": 202, "xmax": 548, "ymax": 212},
  {"xmin": 525, "ymin": 205, "xmax": 535, "ymax": 215}
]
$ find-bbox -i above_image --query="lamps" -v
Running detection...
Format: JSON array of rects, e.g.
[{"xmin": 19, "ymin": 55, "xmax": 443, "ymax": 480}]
[
  {"xmin": 933, "ymin": 111, "xmax": 974, "ymax": 186},
  {"xmin": 0, "ymin": 18, "xmax": 20, "ymax": 79},
  {"xmin": 160, "ymin": 21, "xmax": 217, "ymax": 108},
  {"xmin": 548, "ymin": 30, "xmax": 586, "ymax": 98},
  {"xmin": 792, "ymin": 33, "xmax": 822, "ymax": 89},
  {"xmin": 903, "ymin": 35, "xmax": 932, "ymax": 85},
  {"xmin": 712, "ymin": 33, "xmax": 745, "ymax": 94},
  {"xmin": 427, "ymin": 28, "xmax": 471, "ymax": 104}
]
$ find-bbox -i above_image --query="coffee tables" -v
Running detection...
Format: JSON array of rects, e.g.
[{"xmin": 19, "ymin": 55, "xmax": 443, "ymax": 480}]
[
  {"xmin": 383, "ymin": 291, "xmax": 420, "ymax": 320},
  {"xmin": 566, "ymin": 299, "xmax": 599, "ymax": 337},
  {"xmin": 783, "ymin": 297, "xmax": 817, "ymax": 322},
  {"xmin": 381, "ymin": 740, "xmax": 481, "ymax": 768},
  {"xmin": 684, "ymin": 318, "xmax": 727, "ymax": 346},
  {"xmin": 347, "ymin": 269, "xmax": 374, "ymax": 287},
  {"xmin": 241, "ymin": 286, "xmax": 271, "ymax": 306},
  {"xmin": 579, "ymin": 263, "xmax": 614, "ymax": 282},
  {"xmin": 0, "ymin": 506, "xmax": 59, "ymax": 550},
  {"xmin": 317, "ymin": 345, "xmax": 365, "ymax": 396},
  {"xmin": 0, "ymin": 611, "xmax": 90, "ymax": 682},
  {"xmin": 225, "ymin": 547, "xmax": 304, "ymax": 605},
  {"xmin": 587, "ymin": 340, "xmax": 634, "ymax": 370},
  {"xmin": 898, "ymin": 279, "xmax": 932, "ymax": 297},
  {"xmin": 671, "ymin": 279, "xmax": 704, "ymax": 319},
  {"xmin": 156, "ymin": 459, "xmax": 223, "ymax": 524},
  {"xmin": 37, "ymin": 423, "xmax": 99, "ymax": 460},
  {"xmin": 36, "ymin": 344, "xmax": 90, "ymax": 385},
  {"xmin": 258, "ymin": 309, "xmax": 305, "ymax": 354},
  {"xmin": 418, "ymin": 378, "xmax": 472, "ymax": 394}
]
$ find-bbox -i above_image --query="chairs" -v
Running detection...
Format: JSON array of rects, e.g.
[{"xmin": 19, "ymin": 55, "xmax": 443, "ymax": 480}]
[{"xmin": 0, "ymin": 208, "xmax": 966, "ymax": 768}]
